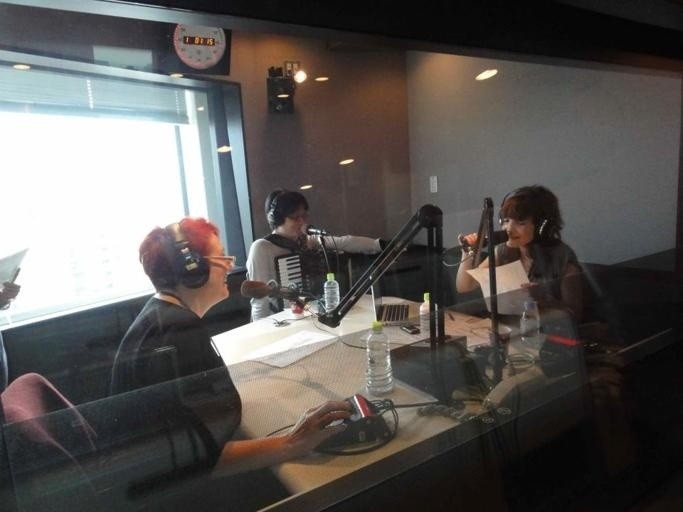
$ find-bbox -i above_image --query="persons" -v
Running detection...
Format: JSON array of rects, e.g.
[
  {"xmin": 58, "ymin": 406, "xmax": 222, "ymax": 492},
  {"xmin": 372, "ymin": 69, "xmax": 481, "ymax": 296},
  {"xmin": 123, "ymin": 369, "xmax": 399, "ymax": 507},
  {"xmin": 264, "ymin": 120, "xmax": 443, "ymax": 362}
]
[
  {"xmin": 0, "ymin": 281, "xmax": 22, "ymax": 395},
  {"xmin": 106, "ymin": 216, "xmax": 352, "ymax": 512},
  {"xmin": 245, "ymin": 187, "xmax": 408, "ymax": 323},
  {"xmin": 455, "ymin": 184, "xmax": 595, "ymax": 329}
]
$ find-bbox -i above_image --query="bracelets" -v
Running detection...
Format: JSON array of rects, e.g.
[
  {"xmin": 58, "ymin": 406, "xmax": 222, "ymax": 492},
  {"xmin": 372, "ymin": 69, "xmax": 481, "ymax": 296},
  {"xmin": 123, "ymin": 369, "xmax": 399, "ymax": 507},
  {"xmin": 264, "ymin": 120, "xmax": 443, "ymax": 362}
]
[{"xmin": 0, "ymin": 302, "xmax": 11, "ymax": 311}]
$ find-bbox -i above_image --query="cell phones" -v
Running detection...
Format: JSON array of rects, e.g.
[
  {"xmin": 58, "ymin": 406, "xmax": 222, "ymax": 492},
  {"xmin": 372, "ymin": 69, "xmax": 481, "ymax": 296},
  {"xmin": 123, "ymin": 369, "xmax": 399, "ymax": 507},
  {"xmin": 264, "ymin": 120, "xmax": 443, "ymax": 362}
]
[{"xmin": 401, "ymin": 324, "xmax": 420, "ymax": 334}]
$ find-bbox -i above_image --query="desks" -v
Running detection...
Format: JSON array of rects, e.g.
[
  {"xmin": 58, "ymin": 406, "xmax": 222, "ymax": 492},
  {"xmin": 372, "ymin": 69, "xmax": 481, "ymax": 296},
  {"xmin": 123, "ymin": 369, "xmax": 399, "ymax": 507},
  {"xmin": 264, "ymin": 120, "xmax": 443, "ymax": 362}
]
[{"xmin": 205, "ymin": 284, "xmax": 600, "ymax": 512}]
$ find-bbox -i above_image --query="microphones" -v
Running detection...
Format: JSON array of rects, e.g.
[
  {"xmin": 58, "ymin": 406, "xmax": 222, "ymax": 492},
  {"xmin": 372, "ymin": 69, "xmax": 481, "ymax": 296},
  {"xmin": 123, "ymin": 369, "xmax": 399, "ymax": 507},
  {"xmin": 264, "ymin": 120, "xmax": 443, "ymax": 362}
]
[
  {"xmin": 300, "ymin": 224, "xmax": 330, "ymax": 237},
  {"xmin": 462, "ymin": 230, "xmax": 509, "ymax": 253},
  {"xmin": 241, "ymin": 280, "xmax": 299, "ymax": 303}
]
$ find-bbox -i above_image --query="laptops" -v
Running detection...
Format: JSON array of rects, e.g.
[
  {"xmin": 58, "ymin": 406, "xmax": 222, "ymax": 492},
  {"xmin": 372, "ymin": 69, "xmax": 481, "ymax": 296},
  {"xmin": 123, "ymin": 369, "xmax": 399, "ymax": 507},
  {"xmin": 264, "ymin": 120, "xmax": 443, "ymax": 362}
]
[{"xmin": 370, "ymin": 276, "xmax": 420, "ymax": 326}]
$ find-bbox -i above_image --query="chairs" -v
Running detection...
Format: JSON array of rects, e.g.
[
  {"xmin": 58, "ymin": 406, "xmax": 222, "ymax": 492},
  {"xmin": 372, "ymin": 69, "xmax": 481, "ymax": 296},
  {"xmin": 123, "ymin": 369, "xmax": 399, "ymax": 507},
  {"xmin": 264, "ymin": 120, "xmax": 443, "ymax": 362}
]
[
  {"xmin": 1, "ymin": 367, "xmax": 100, "ymax": 512},
  {"xmin": 575, "ymin": 259, "xmax": 680, "ymax": 497}
]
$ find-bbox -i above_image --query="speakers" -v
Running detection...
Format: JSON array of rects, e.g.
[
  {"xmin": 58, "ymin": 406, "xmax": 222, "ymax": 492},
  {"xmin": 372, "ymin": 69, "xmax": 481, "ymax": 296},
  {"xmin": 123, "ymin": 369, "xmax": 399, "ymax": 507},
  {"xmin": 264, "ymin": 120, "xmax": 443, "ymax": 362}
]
[{"xmin": 267, "ymin": 73, "xmax": 294, "ymax": 115}]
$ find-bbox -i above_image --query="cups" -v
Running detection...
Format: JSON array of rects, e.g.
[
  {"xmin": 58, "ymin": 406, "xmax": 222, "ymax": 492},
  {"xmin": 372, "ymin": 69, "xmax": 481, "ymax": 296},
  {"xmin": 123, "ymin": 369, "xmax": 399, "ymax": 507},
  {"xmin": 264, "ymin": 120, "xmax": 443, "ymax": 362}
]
[{"xmin": 490, "ymin": 327, "xmax": 511, "ymax": 356}]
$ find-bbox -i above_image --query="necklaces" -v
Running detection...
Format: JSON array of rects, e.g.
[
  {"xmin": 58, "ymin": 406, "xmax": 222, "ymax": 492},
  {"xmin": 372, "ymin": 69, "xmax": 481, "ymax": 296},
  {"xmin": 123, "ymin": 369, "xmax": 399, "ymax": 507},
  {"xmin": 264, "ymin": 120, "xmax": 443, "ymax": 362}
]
[{"xmin": 158, "ymin": 290, "xmax": 222, "ymax": 358}]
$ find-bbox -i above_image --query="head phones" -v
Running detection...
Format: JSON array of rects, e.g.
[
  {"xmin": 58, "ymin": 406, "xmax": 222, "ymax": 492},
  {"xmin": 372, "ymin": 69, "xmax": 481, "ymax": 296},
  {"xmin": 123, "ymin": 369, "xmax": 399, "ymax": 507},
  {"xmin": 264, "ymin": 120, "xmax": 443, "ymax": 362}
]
[
  {"xmin": 167, "ymin": 223, "xmax": 209, "ymax": 289},
  {"xmin": 266, "ymin": 191, "xmax": 291, "ymax": 230},
  {"xmin": 498, "ymin": 187, "xmax": 552, "ymax": 238}
]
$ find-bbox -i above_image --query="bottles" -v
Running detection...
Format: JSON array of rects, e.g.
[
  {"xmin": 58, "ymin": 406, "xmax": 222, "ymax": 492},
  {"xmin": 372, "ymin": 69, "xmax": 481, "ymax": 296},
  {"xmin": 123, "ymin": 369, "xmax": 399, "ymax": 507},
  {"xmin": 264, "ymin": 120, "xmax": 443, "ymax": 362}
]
[
  {"xmin": 367, "ymin": 322, "xmax": 393, "ymax": 396},
  {"xmin": 520, "ymin": 300, "xmax": 542, "ymax": 352},
  {"xmin": 419, "ymin": 293, "xmax": 439, "ymax": 336},
  {"xmin": 324, "ymin": 273, "xmax": 341, "ymax": 312}
]
